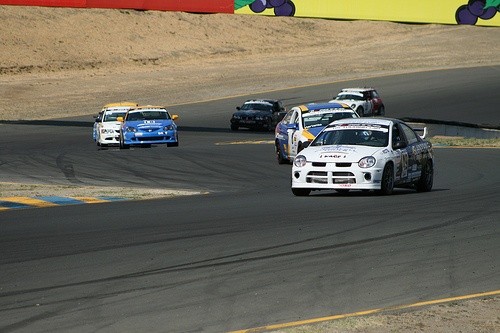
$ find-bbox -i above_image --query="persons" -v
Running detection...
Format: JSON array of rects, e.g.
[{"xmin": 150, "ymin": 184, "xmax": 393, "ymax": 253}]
[{"xmin": 360, "ymin": 130, "xmax": 377, "ymax": 144}]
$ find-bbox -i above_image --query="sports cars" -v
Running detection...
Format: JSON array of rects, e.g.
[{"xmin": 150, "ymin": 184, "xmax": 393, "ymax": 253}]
[
  {"xmin": 329, "ymin": 87, "xmax": 385, "ymax": 118},
  {"xmin": 273, "ymin": 101, "xmax": 435, "ymax": 197},
  {"xmin": 92, "ymin": 101, "xmax": 180, "ymax": 150},
  {"xmin": 230, "ymin": 98, "xmax": 287, "ymax": 132}
]
